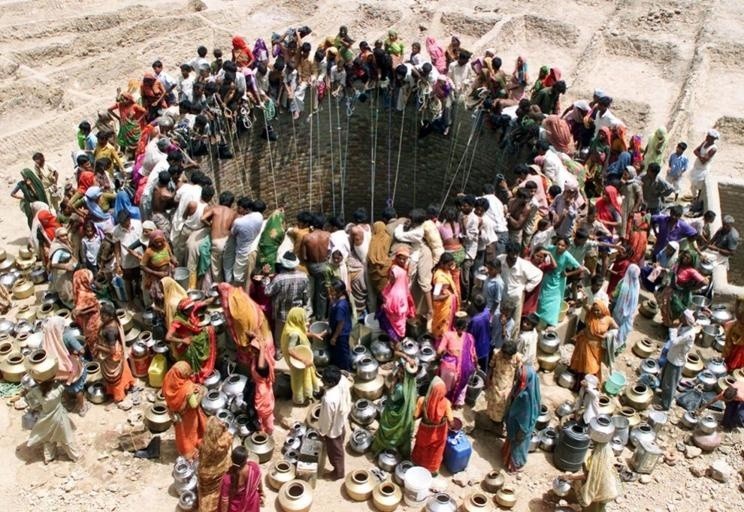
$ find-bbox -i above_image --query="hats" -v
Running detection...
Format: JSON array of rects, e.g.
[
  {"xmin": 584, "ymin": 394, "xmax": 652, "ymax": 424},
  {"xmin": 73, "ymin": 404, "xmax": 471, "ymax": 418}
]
[
  {"xmin": 594, "ymin": 88, "xmax": 604, "ymax": 99},
  {"xmin": 455, "ymin": 311, "xmax": 468, "ymax": 318}
]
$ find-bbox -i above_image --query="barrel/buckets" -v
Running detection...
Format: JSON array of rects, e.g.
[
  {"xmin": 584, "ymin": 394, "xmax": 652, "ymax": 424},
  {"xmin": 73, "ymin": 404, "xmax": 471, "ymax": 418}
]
[
  {"xmin": 559, "ymin": 301, "xmax": 569, "ymax": 322},
  {"xmin": 128, "ymin": 347, "xmax": 151, "ymax": 377},
  {"xmin": 112, "ymin": 273, "xmax": 137, "ymax": 302},
  {"xmin": 403, "ymin": 466, "xmax": 432, "ymax": 506},
  {"xmin": 695, "ymin": 325, "xmax": 720, "ymax": 348},
  {"xmin": 147, "ymin": 354, "xmax": 168, "ymax": 388},
  {"xmin": 442, "ymin": 430, "xmax": 472, "ymax": 473},
  {"xmin": 690, "ymin": 295, "xmax": 712, "ymax": 310}
]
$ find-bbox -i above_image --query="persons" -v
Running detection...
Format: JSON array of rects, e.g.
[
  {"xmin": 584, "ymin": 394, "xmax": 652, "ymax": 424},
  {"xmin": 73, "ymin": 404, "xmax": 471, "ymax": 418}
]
[
  {"xmin": 270, "ymin": 31, "xmax": 288, "ymax": 76},
  {"xmin": 693, "ymin": 375, "xmax": 744, "ymax": 435},
  {"xmin": 153, "ymin": 172, "xmax": 175, "ymax": 242},
  {"xmin": 557, "ymin": 432, "xmax": 625, "ymax": 508},
  {"xmin": 383, "ymin": 49, "xmax": 399, "ymax": 79},
  {"xmin": 455, "ymin": 195, "xmax": 464, "ymax": 205},
  {"xmin": 325, "ymin": 280, "xmax": 353, "ymax": 371},
  {"xmin": 77, "ymin": 121, "xmax": 98, "ymax": 162},
  {"xmin": 496, "ymin": 242, "xmax": 542, "ymax": 338},
  {"xmin": 287, "ymin": 212, "xmax": 312, "ymax": 263},
  {"xmin": 250, "ymin": 359, "xmax": 276, "ymax": 434},
  {"xmin": 485, "ymin": 98, "xmax": 519, "ymax": 114},
  {"xmin": 427, "ymin": 202, "xmax": 448, "ymax": 230},
  {"xmin": 355, "ymin": 208, "xmax": 374, "ymax": 240},
  {"xmin": 316, "ymin": 365, "xmax": 355, "ymax": 480},
  {"xmin": 657, "ymin": 310, "xmax": 694, "ymax": 409},
  {"xmin": 491, "ymin": 104, "xmax": 527, "ymax": 130},
  {"xmin": 380, "ymin": 208, "xmax": 409, "ymax": 257},
  {"xmin": 201, "ymin": 190, "xmax": 238, "ymax": 282},
  {"xmin": 71, "ymin": 186, "xmax": 118, "ymax": 234},
  {"xmin": 98, "ymin": 228, "xmax": 128, "ymax": 304},
  {"xmin": 514, "ymin": 98, "xmax": 531, "ymax": 118},
  {"xmin": 190, "ymin": 46, "xmax": 211, "ymax": 80},
  {"xmin": 164, "ymin": 99, "xmax": 189, "ymax": 126},
  {"xmin": 509, "ymin": 54, "xmax": 528, "ymax": 101},
  {"xmin": 175, "ymin": 171, "xmax": 204, "ymax": 201},
  {"xmin": 586, "ymin": 96, "xmax": 623, "ymax": 137},
  {"xmin": 655, "ymin": 248, "xmax": 704, "ymax": 327},
  {"xmin": 522, "ymin": 244, "xmax": 556, "ymax": 322},
  {"xmin": 31, "ymin": 373, "xmax": 78, "ymax": 464},
  {"xmin": 188, "ymin": 223, "xmax": 215, "ymax": 277},
  {"xmin": 498, "ymin": 177, "xmax": 531, "ymax": 253},
  {"xmin": 549, "ymin": 179, "xmax": 577, "ymax": 222},
  {"xmin": 415, "ymin": 207, "xmax": 445, "ymax": 268},
  {"xmin": 94, "ymin": 156, "xmax": 113, "ymax": 194},
  {"xmin": 613, "ymin": 263, "xmax": 642, "ymax": 353},
  {"xmin": 229, "ymin": 37, "xmax": 253, "ymax": 67},
  {"xmin": 134, "ymin": 117, "xmax": 173, "ymax": 170},
  {"xmin": 93, "ymin": 305, "xmax": 136, "ymax": 401},
  {"xmin": 232, "ymin": 199, "xmax": 263, "ymax": 290},
  {"xmin": 73, "ymin": 268, "xmax": 101, "ymax": 338},
  {"xmin": 414, "ymin": 376, "xmax": 454, "ymax": 480},
  {"xmin": 426, "ymin": 253, "xmax": 458, "ymax": 345},
  {"xmin": 637, "ymin": 163, "xmax": 678, "ymax": 212},
  {"xmin": 373, "ymin": 38, "xmax": 384, "ymax": 56},
  {"xmin": 513, "ymin": 163, "xmax": 547, "ymax": 219},
  {"xmin": 195, "ymin": 418, "xmax": 233, "ymax": 512},
  {"xmin": 385, "ymin": 29, "xmax": 404, "ymax": 55},
  {"xmin": 575, "ymin": 273, "xmax": 609, "ymax": 344},
  {"xmin": 372, "ymin": 351, "xmax": 416, "ymax": 457},
  {"xmin": 157, "ymin": 276, "xmax": 193, "ymax": 354},
  {"xmin": 330, "ymin": 59, "xmax": 346, "ymax": 97},
  {"xmin": 474, "ymin": 198, "xmax": 496, "ymax": 257},
  {"xmin": 167, "ymin": 165, "xmax": 183, "ymax": 186},
  {"xmin": 426, "ymin": 34, "xmax": 443, "ymax": 64},
  {"xmin": 555, "ymin": 200, "xmax": 579, "ymax": 239},
  {"xmin": 702, "ymin": 244, "xmax": 732, "ymax": 276},
  {"xmin": 329, "ymin": 212, "xmax": 350, "ymax": 267},
  {"xmin": 609, "ymin": 241, "xmax": 631, "ymax": 292},
  {"xmin": 533, "ymin": 67, "xmax": 564, "ymax": 115},
  {"xmin": 628, "ymin": 133, "xmax": 643, "ymax": 164},
  {"xmin": 107, "ymin": 92, "xmax": 147, "ymax": 154},
  {"xmin": 577, "ymin": 375, "xmax": 601, "ymax": 425},
  {"xmin": 393, "ymin": 247, "xmax": 416, "ymax": 292},
  {"xmin": 503, "ymin": 366, "xmax": 540, "ymax": 472},
  {"xmin": 152, "ymin": 60, "xmax": 177, "ymax": 108},
  {"xmin": 178, "ymin": 61, "xmax": 194, "ymax": 96},
  {"xmin": 226, "ymin": 87, "xmax": 244, "ymax": 139},
  {"xmin": 449, "ymin": 48, "xmax": 462, "ymax": 60},
  {"xmin": 110, "ymin": 208, "xmax": 145, "ymax": 298},
  {"xmin": 190, "ymin": 296, "xmax": 218, "ymax": 379},
  {"xmin": 204, "ymin": 79, "xmax": 224, "ymax": 146},
  {"xmin": 690, "ymin": 128, "xmax": 719, "ymax": 197},
  {"xmin": 380, "ymin": 264, "xmax": 412, "ymax": 337},
  {"xmin": 221, "ymin": 446, "xmax": 264, "ymax": 512},
  {"xmin": 211, "ymin": 48, "xmax": 226, "ymax": 75},
  {"xmin": 196, "ymin": 63, "xmax": 214, "ymax": 88},
  {"xmin": 610, "ymin": 125, "xmax": 626, "ymax": 158},
  {"xmin": 531, "ymin": 150, "xmax": 579, "ymax": 186},
  {"xmin": 340, "ymin": 45, "xmax": 353, "ymax": 61},
  {"xmin": 94, "ymin": 110, "xmax": 117, "ymax": 144},
  {"xmin": 545, "ymin": 184, "xmax": 558, "ymax": 203},
  {"xmin": 37, "ymin": 212, "xmax": 63, "ymax": 275},
  {"xmin": 328, "ymin": 249, "xmax": 351, "ymax": 287},
  {"xmin": 492, "ymin": 300, "xmax": 516, "ymax": 352},
  {"xmin": 301, "ymin": 214, "xmax": 332, "ymax": 322},
  {"xmin": 365, "ymin": 53, "xmax": 377, "ymax": 78},
  {"xmin": 547, "ymin": 145, "xmax": 587, "ymax": 179},
  {"xmin": 413, "ymin": 61, "xmax": 437, "ymax": 92},
  {"xmin": 625, "ymin": 208, "xmax": 656, "ymax": 261},
  {"xmin": 644, "ymin": 125, "xmax": 666, "ymax": 172},
  {"xmin": 335, "ymin": 25, "xmax": 353, "ymax": 46},
  {"xmin": 483, "ymin": 87, "xmax": 506, "ymax": 107},
  {"xmin": 531, "ymin": 214, "xmax": 565, "ymax": 254},
  {"xmin": 396, "ymin": 60, "xmax": 419, "ymax": 90},
  {"xmin": 588, "ymin": 86, "xmax": 601, "ymax": 111},
  {"xmin": 241, "ymin": 65, "xmax": 265, "ymax": 116},
  {"xmin": 399, "ymin": 207, "xmax": 435, "ymax": 319},
  {"xmin": 651, "ymin": 204, "xmax": 697, "ymax": 261},
  {"xmin": 189, "ymin": 80, "xmax": 207, "ymax": 112},
  {"xmin": 282, "ymin": 27, "xmax": 302, "ymax": 69},
  {"xmin": 706, "ymin": 215, "xmax": 741, "ymax": 260},
  {"xmin": 666, "ymin": 142, "xmax": 689, "ymax": 192},
  {"xmin": 137, "ymin": 138, "xmax": 170, "ymax": 196},
  {"xmin": 410, "ymin": 43, "xmax": 427, "ymax": 67},
  {"xmin": 724, "ymin": 297, "xmax": 743, "ymax": 375},
  {"xmin": 31, "ymin": 199, "xmax": 55, "ymax": 256},
  {"xmin": 77, "ymin": 219, "xmax": 102, "ymax": 273},
  {"xmin": 182, "ymin": 110, "xmax": 212, "ymax": 146},
  {"xmin": 292, "ymin": 38, "xmax": 314, "ymax": 119},
  {"xmin": 175, "ymin": 186, "xmax": 219, "ymax": 275},
  {"xmin": 594, "ymin": 186, "xmax": 624, "ymax": 232},
  {"xmin": 32, "ymin": 153, "xmax": 60, "ymax": 214},
  {"xmin": 515, "ymin": 313, "xmax": 540, "ymax": 373},
  {"xmin": 49, "ymin": 228, "xmax": 72, "ymax": 266},
  {"xmin": 75, "ymin": 156, "xmax": 92, "ymax": 191},
  {"xmin": 218, "ymin": 57, "xmax": 246, "ymax": 101},
  {"xmin": 139, "ymin": 73, "xmax": 169, "ymax": 119},
  {"xmin": 433, "ymin": 75, "xmax": 455, "ymax": 135},
  {"xmin": 583, "ymin": 178, "xmax": 605, "ymax": 195},
  {"xmin": 533, "ymin": 79, "xmax": 567, "ymax": 116},
  {"xmin": 472, "ymin": 46, "xmax": 496, "ymax": 79},
  {"xmin": 164, "ymin": 298, "xmax": 206, "ymax": 386},
  {"xmin": 466, "ymin": 297, "xmax": 499, "ymax": 363},
  {"xmin": 529, "ymin": 65, "xmax": 549, "ymax": 98},
  {"xmin": 252, "ymin": 62, "xmax": 270, "ymax": 100},
  {"xmin": 368, "ymin": 219, "xmax": 394, "ymax": 292},
  {"xmin": 216, "ymin": 74, "xmax": 235, "ymax": 104},
  {"xmin": 445, "ymin": 34, "xmax": 462, "ymax": 57},
  {"xmin": 349, "ymin": 57, "xmax": 369, "ymax": 94},
  {"xmin": 217, "ymin": 283, "xmax": 273, "ymax": 363},
  {"xmin": 257, "ymin": 206, "xmax": 286, "ymax": 279},
  {"xmin": 143, "ymin": 233, "xmax": 179, "ymax": 303},
  {"xmin": 576, "ymin": 202, "xmax": 609, "ymax": 247},
  {"xmin": 104, "ymin": 130, "xmax": 121, "ymax": 150},
  {"xmin": 324, "ymin": 48, "xmax": 340, "ymax": 62},
  {"xmin": 174, "ymin": 173, "xmax": 212, "ymax": 251},
  {"xmin": 526, "ymin": 165, "xmax": 550, "ymax": 190},
  {"xmin": 93, "ymin": 131, "xmax": 126, "ymax": 191},
  {"xmin": 562, "ymin": 100, "xmax": 590, "ymax": 136},
  {"xmin": 442, "ymin": 208, "xmax": 465, "ymax": 263},
  {"xmin": 252, "ymin": 37, "xmax": 269, "ymax": 63},
  {"xmin": 161, "ymin": 360, "xmax": 208, "ymax": 458},
  {"xmin": 437, "ymin": 313, "xmax": 478, "ymax": 408},
  {"xmin": 582, "ymin": 128, "xmax": 609, "ymax": 178},
  {"xmin": 476, "ymin": 257, "xmax": 500, "ymax": 318},
  {"xmin": 486, "ymin": 56, "xmax": 507, "ymax": 93},
  {"xmin": 346, "ymin": 256, "xmax": 368, "ymax": 319},
  {"xmin": 49, "ymin": 254, "xmax": 81, "ymax": 310},
  {"xmin": 485, "ymin": 341, "xmax": 521, "ymax": 435},
  {"xmin": 349, "ymin": 227, "xmax": 373, "ymax": 265},
  {"xmin": 457, "ymin": 193, "xmax": 478, "ymax": 299},
  {"xmin": 528, "ymin": 102, "xmax": 544, "ymax": 120},
  {"xmin": 79, "ymin": 119, "xmax": 95, "ymax": 163},
  {"xmin": 281, "ymin": 307, "xmax": 323, "ymax": 405},
  {"xmin": 129, "ymin": 223, "xmax": 129, "ymax": 225},
  {"xmin": 282, "ymin": 59, "xmax": 296, "ymax": 106},
  {"xmin": 225, "ymin": 196, "xmax": 265, "ymax": 284},
  {"xmin": 129, "ymin": 221, "xmax": 165, "ymax": 286},
  {"xmin": 134, "ymin": 152, "xmax": 183, "ymax": 214},
  {"xmin": 261, "ymin": 251, "xmax": 309, "ymax": 359},
  {"xmin": 125, "ymin": 80, "xmax": 147, "ymax": 106},
  {"xmin": 449, "ymin": 50, "xmax": 472, "ymax": 96},
  {"xmin": 312, "ymin": 48, "xmax": 328, "ymax": 113},
  {"xmin": 10, "ymin": 168, "xmax": 48, "ymax": 234},
  {"xmin": 607, "ymin": 153, "xmax": 633, "ymax": 175},
  {"xmin": 652, "ymin": 240, "xmax": 678, "ymax": 270},
  {"xmin": 477, "ymin": 181, "xmax": 512, "ymax": 252},
  {"xmin": 43, "ymin": 313, "xmax": 91, "ymax": 417},
  {"xmin": 614, "ymin": 167, "xmax": 641, "ymax": 239},
  {"xmin": 483, "ymin": 56, "xmax": 495, "ymax": 89},
  {"xmin": 269, "ymin": 62, "xmax": 283, "ymax": 109},
  {"xmin": 586, "ymin": 245, "xmax": 610, "ymax": 277},
  {"xmin": 689, "ymin": 211, "xmax": 715, "ymax": 244},
  {"xmin": 316, "ymin": 36, "xmax": 333, "ymax": 50},
  {"xmin": 539, "ymin": 238, "xmax": 584, "ymax": 329},
  {"xmin": 526, "ymin": 179, "xmax": 542, "ymax": 249},
  {"xmin": 520, "ymin": 117, "xmax": 544, "ymax": 144},
  {"xmin": 177, "ymin": 96, "xmax": 190, "ymax": 107},
  {"xmin": 570, "ymin": 301, "xmax": 617, "ymax": 389},
  {"xmin": 357, "ymin": 41, "xmax": 376, "ymax": 63},
  {"xmin": 155, "ymin": 129, "xmax": 196, "ymax": 167},
  {"xmin": 243, "ymin": 334, "xmax": 280, "ymax": 361},
  {"xmin": 479, "ymin": 67, "xmax": 501, "ymax": 103}
]
